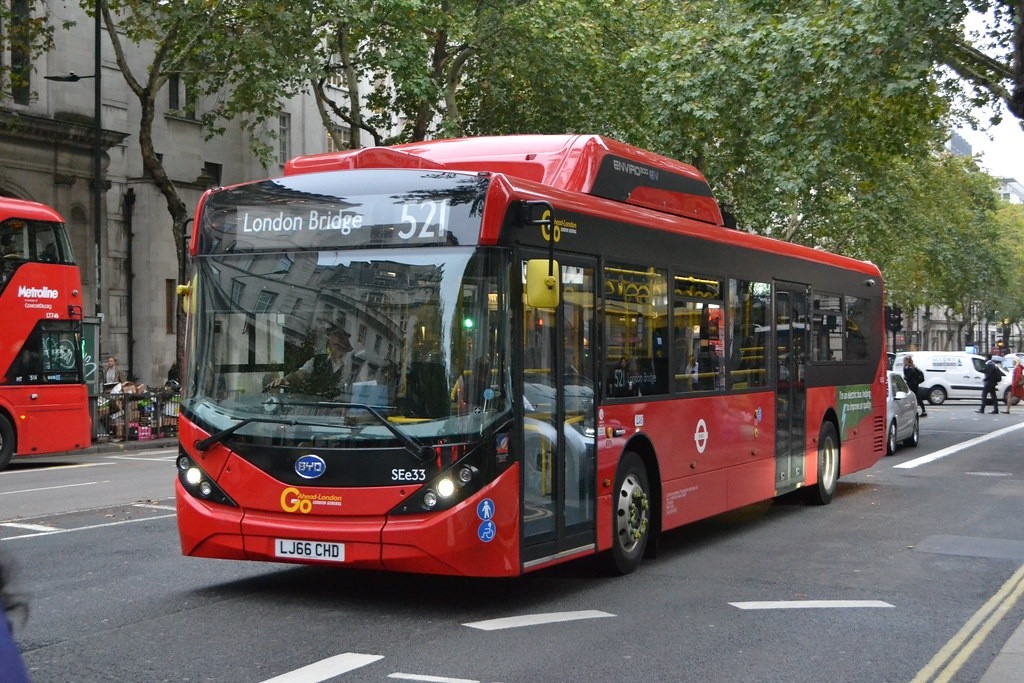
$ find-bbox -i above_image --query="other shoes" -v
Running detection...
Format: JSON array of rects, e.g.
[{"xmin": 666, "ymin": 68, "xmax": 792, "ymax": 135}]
[
  {"xmin": 1000, "ymin": 410, "xmax": 1010, "ymax": 414},
  {"xmin": 989, "ymin": 409, "xmax": 999, "ymax": 414},
  {"xmin": 974, "ymin": 410, "xmax": 985, "ymax": 414},
  {"xmin": 919, "ymin": 413, "xmax": 928, "ymax": 417}
]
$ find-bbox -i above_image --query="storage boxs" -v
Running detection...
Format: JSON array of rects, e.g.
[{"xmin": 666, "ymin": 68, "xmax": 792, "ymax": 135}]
[{"xmin": 98, "ymin": 380, "xmax": 183, "ymax": 441}]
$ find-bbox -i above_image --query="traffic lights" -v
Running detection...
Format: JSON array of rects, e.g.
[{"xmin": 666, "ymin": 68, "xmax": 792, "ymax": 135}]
[
  {"xmin": 538, "ymin": 317, "xmax": 543, "ymax": 330},
  {"xmin": 885, "ymin": 305, "xmax": 904, "ymax": 332},
  {"xmin": 463, "ymin": 318, "xmax": 473, "ymax": 329}
]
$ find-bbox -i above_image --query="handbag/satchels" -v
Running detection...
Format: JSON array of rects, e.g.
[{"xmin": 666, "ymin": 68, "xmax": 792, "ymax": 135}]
[{"xmin": 1013, "ymin": 374, "xmax": 1024, "ymax": 386}]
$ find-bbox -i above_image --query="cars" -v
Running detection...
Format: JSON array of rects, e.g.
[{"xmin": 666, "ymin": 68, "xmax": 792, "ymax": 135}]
[
  {"xmin": 886, "ymin": 369, "xmax": 919, "ymax": 456},
  {"xmin": 1001, "ymin": 352, "xmax": 1024, "ymax": 374}
]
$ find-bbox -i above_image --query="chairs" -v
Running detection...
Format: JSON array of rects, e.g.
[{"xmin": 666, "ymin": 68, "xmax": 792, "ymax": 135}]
[{"xmin": 608, "ymin": 352, "xmax": 722, "ymax": 395}]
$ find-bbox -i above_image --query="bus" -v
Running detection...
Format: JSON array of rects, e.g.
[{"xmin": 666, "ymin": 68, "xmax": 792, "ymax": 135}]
[
  {"xmin": 171, "ymin": 127, "xmax": 895, "ymax": 581},
  {"xmin": 0, "ymin": 195, "xmax": 92, "ymax": 473}
]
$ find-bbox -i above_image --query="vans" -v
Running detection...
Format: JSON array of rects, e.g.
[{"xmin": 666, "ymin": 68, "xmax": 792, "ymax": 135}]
[{"xmin": 892, "ymin": 350, "xmax": 1022, "ymax": 406}]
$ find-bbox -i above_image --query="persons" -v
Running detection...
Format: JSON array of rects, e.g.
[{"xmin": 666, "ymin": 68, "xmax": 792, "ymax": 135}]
[
  {"xmin": 0, "ymin": 549, "xmax": 32, "ymax": 683},
  {"xmin": 104, "ymin": 354, "xmax": 126, "ymax": 383},
  {"xmin": 611, "ymin": 357, "xmax": 656, "ymax": 396},
  {"xmin": 463, "ymin": 356, "xmax": 498, "ymax": 407},
  {"xmin": 990, "ymin": 344, "xmax": 997, "ymax": 355},
  {"xmin": 684, "ymin": 354, "xmax": 701, "ymax": 390},
  {"xmin": 165, "ymin": 370, "xmax": 179, "ymax": 387},
  {"xmin": 977, "ymin": 353, "xmax": 998, "ymax": 414},
  {"xmin": 903, "ymin": 356, "xmax": 927, "ymax": 417},
  {"xmin": 267, "ymin": 328, "xmax": 368, "ymax": 398},
  {"xmin": 1001, "ymin": 356, "xmax": 1024, "ymax": 414},
  {"xmin": 902, "ymin": 340, "xmax": 917, "ymax": 351},
  {"xmin": 39, "ymin": 243, "xmax": 58, "ymax": 262}
]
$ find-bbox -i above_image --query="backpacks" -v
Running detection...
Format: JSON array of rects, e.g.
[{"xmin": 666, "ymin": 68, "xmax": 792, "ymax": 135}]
[
  {"xmin": 995, "ymin": 365, "xmax": 1002, "ymax": 383},
  {"xmin": 911, "ymin": 365, "xmax": 924, "ymax": 383}
]
